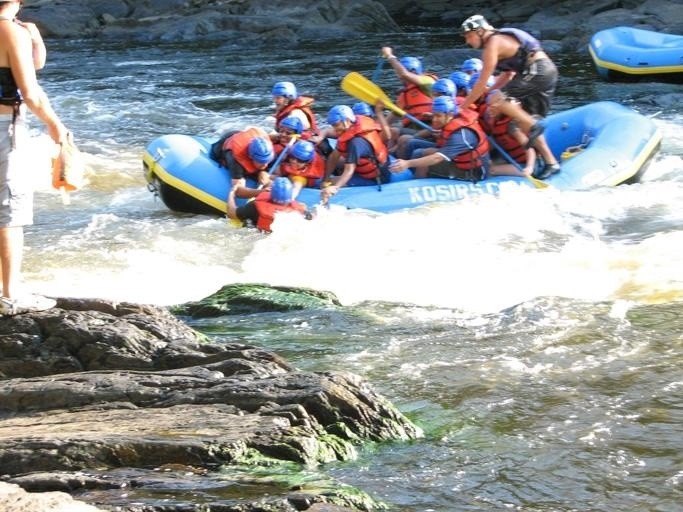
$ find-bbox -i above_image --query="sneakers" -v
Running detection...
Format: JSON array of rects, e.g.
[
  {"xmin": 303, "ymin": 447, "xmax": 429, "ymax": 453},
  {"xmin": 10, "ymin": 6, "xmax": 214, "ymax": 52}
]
[
  {"xmin": 535, "ymin": 162, "xmax": 561, "ymax": 179},
  {"xmin": 526, "ymin": 120, "xmax": 545, "ymax": 150},
  {"xmin": 0, "ymin": 294, "xmax": 58, "ymax": 317}
]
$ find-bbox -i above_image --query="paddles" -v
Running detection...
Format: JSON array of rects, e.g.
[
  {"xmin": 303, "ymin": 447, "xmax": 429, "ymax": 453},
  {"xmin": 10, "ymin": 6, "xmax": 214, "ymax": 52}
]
[
  {"xmin": 229, "ymin": 134, "xmax": 301, "ymax": 229},
  {"xmin": 487, "ymin": 135, "xmax": 549, "ymax": 189},
  {"xmin": 341, "ymin": 72, "xmax": 434, "ymax": 132}
]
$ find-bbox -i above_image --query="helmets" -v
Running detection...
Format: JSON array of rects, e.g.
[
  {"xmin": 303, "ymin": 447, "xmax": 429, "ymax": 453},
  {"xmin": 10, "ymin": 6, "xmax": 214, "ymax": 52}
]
[
  {"xmin": 288, "ymin": 139, "xmax": 315, "ymax": 164},
  {"xmin": 432, "ymin": 95, "xmax": 458, "ymax": 116},
  {"xmin": 351, "ymin": 102, "xmax": 375, "ymax": 118},
  {"xmin": 432, "ymin": 58, "xmax": 496, "ymax": 99},
  {"xmin": 271, "ymin": 82, "xmax": 298, "ymax": 100},
  {"xmin": 326, "ymin": 105, "xmax": 356, "ymax": 124},
  {"xmin": 279, "ymin": 116, "xmax": 304, "ymax": 134},
  {"xmin": 271, "ymin": 176, "xmax": 293, "ymax": 204},
  {"xmin": 248, "ymin": 136, "xmax": 273, "ymax": 164},
  {"xmin": 399, "ymin": 57, "xmax": 423, "ymax": 74},
  {"xmin": 460, "ymin": 14, "xmax": 495, "ymax": 33}
]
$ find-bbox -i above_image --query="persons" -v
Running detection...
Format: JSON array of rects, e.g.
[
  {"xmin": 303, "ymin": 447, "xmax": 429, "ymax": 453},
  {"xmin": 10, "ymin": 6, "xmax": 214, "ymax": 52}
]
[
  {"xmin": 208, "ymin": 14, "xmax": 562, "ymax": 235},
  {"xmin": 1, "ymin": 1, "xmax": 71, "ymax": 317}
]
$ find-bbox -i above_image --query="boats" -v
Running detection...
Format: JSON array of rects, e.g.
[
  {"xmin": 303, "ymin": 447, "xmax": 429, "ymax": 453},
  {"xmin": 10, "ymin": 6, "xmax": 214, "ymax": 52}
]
[
  {"xmin": 588, "ymin": 24, "xmax": 682, "ymax": 82},
  {"xmin": 138, "ymin": 101, "xmax": 664, "ymax": 224}
]
